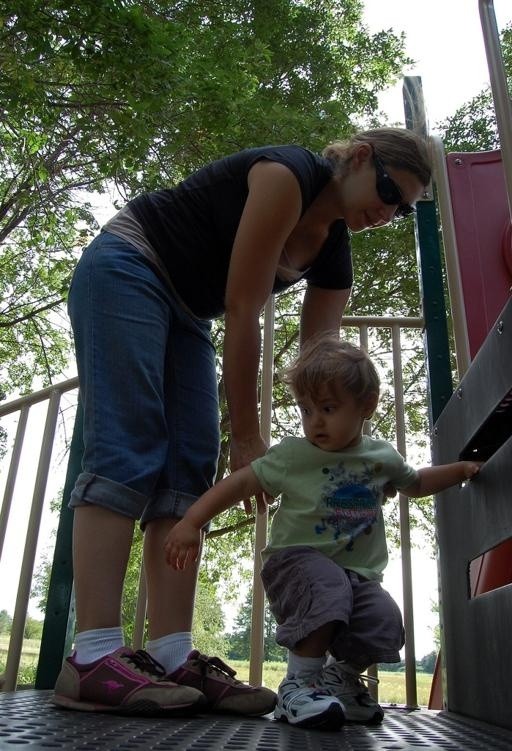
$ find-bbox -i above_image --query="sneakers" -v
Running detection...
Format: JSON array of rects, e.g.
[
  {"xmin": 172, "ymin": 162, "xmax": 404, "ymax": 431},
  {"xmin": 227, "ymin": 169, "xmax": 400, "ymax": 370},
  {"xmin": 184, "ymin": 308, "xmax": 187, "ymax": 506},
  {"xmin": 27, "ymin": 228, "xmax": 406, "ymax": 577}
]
[
  {"xmin": 275, "ymin": 670, "xmax": 346, "ymax": 728},
  {"xmin": 51, "ymin": 645, "xmax": 207, "ymax": 716},
  {"xmin": 166, "ymin": 649, "xmax": 278, "ymax": 717},
  {"xmin": 324, "ymin": 659, "xmax": 385, "ymax": 725}
]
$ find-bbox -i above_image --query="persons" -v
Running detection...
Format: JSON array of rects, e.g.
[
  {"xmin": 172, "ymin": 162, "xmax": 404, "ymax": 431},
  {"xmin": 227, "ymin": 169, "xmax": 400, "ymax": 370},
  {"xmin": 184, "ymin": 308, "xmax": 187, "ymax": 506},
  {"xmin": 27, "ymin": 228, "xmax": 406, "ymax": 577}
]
[
  {"xmin": 160, "ymin": 334, "xmax": 485, "ymax": 729},
  {"xmin": 49, "ymin": 126, "xmax": 438, "ymax": 719}
]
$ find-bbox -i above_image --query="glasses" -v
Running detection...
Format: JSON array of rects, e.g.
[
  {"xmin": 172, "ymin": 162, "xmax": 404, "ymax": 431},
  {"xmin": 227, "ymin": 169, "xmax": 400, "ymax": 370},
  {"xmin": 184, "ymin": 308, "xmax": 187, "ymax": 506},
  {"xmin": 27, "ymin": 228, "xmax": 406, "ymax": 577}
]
[{"xmin": 372, "ymin": 146, "xmax": 414, "ymax": 220}]
